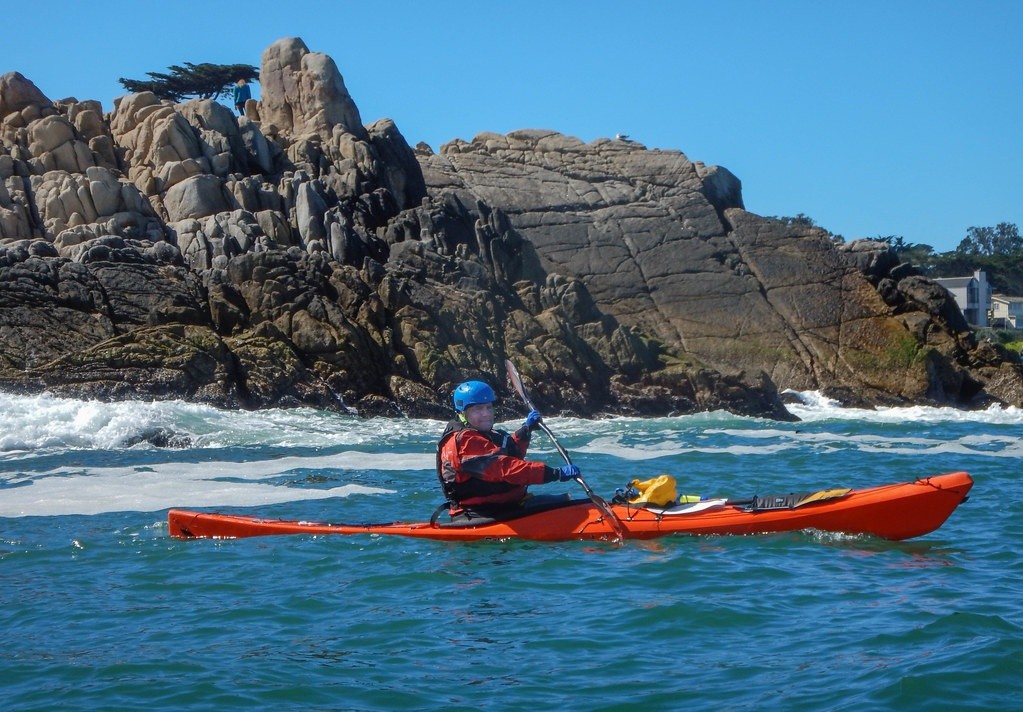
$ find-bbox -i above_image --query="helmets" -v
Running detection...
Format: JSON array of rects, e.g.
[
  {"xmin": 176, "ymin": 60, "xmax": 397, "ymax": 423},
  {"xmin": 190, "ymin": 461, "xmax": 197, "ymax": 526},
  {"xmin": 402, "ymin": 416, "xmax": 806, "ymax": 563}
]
[{"xmin": 453, "ymin": 381, "xmax": 497, "ymax": 411}]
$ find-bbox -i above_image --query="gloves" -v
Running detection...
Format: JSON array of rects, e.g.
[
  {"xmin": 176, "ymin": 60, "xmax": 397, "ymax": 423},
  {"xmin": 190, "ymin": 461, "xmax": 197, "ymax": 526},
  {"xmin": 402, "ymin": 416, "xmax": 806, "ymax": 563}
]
[
  {"xmin": 525, "ymin": 410, "xmax": 544, "ymax": 431},
  {"xmin": 556, "ymin": 464, "xmax": 580, "ymax": 482}
]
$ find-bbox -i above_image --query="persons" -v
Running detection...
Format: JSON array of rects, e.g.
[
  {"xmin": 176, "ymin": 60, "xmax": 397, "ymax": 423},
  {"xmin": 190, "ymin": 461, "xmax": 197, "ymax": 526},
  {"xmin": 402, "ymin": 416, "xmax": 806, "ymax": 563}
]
[
  {"xmin": 435, "ymin": 380, "xmax": 581, "ymax": 524},
  {"xmin": 234, "ymin": 79, "xmax": 252, "ymax": 116}
]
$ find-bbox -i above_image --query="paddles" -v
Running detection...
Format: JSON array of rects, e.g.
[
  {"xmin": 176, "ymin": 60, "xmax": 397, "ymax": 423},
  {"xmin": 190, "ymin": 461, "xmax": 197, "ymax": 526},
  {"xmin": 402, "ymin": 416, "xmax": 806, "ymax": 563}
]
[{"xmin": 501, "ymin": 357, "xmax": 634, "ymax": 540}]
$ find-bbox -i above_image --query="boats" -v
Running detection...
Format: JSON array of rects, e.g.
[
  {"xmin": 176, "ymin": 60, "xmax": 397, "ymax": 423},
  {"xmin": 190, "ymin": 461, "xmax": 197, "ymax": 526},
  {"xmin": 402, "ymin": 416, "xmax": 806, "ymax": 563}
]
[{"xmin": 168, "ymin": 471, "xmax": 979, "ymax": 543}]
